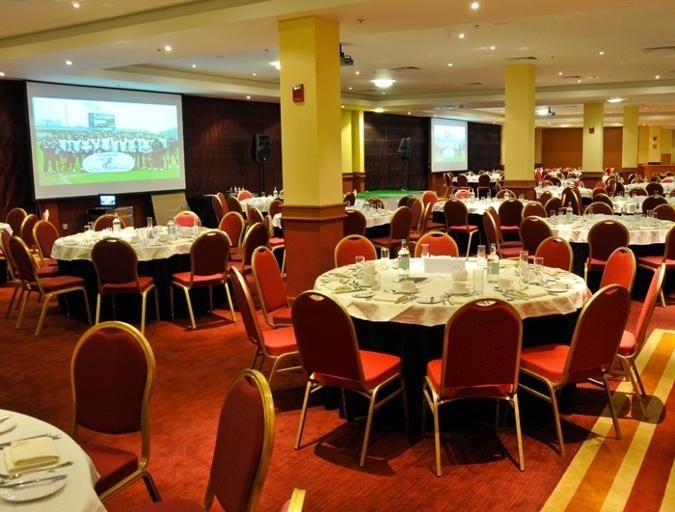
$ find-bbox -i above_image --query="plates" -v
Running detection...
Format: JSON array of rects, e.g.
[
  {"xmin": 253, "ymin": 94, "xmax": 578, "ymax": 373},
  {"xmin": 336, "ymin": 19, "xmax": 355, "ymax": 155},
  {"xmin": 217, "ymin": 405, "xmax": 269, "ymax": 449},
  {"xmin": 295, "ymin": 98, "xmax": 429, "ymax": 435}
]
[
  {"xmin": 0, "ymin": 464, "xmax": 67, "ymax": 503},
  {"xmin": 0, "ymin": 411, "xmax": 17, "ymax": 433},
  {"xmin": 352, "ymin": 290, "xmax": 377, "ymax": 298},
  {"xmin": 548, "ymin": 287, "xmax": 568, "ymax": 293},
  {"xmin": 416, "ymin": 295, "xmax": 441, "ymax": 304}
]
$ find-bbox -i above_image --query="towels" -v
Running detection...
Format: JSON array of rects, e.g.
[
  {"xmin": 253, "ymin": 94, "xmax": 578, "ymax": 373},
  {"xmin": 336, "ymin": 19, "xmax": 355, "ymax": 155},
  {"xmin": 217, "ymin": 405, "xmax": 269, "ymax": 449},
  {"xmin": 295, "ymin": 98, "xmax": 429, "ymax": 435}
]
[
  {"xmin": 325, "ymin": 281, "xmax": 352, "ymax": 292},
  {"xmin": 3, "ymin": 435, "xmax": 60, "ymax": 474},
  {"xmin": 499, "ymin": 257, "xmax": 518, "ymax": 266},
  {"xmin": 448, "ymin": 294, "xmax": 471, "ymax": 306},
  {"xmin": 512, "ymin": 288, "xmax": 548, "ymax": 299},
  {"xmin": 329, "ymin": 267, "xmax": 352, "ymax": 277},
  {"xmin": 543, "ymin": 266, "xmax": 570, "ymax": 278},
  {"xmin": 374, "ymin": 291, "xmax": 408, "ymax": 304},
  {"xmin": 63, "ymin": 237, "xmax": 78, "ymax": 246}
]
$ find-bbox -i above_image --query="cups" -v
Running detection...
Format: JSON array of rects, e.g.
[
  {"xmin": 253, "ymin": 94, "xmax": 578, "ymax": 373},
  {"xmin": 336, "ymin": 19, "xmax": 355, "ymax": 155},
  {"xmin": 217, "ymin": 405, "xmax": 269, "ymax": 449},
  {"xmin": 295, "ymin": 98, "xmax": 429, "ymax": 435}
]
[
  {"xmin": 621, "ymin": 208, "xmax": 627, "ymax": 216},
  {"xmin": 473, "ymin": 269, "xmax": 484, "ymax": 295},
  {"xmin": 477, "ymin": 245, "xmax": 486, "ymax": 267},
  {"xmin": 355, "ymin": 256, "xmax": 365, "ymax": 279},
  {"xmin": 550, "ymin": 210, "xmax": 555, "ymax": 218},
  {"xmin": 558, "ymin": 208, "xmax": 563, "ymax": 217},
  {"xmin": 497, "ymin": 267, "xmax": 515, "ymax": 291},
  {"xmin": 381, "ymin": 249, "xmax": 389, "ymax": 269},
  {"xmin": 520, "ymin": 266, "xmax": 529, "ymax": 289},
  {"xmin": 421, "ymin": 244, "xmax": 430, "ymax": 259},
  {"xmin": 83, "ymin": 226, "xmax": 90, "ymax": 242},
  {"xmin": 647, "ymin": 210, "xmax": 653, "ymax": 218},
  {"xmin": 481, "ymin": 197, "xmax": 485, "ymax": 204},
  {"xmin": 519, "ymin": 251, "xmax": 529, "ymax": 266},
  {"xmin": 534, "ymin": 257, "xmax": 543, "ymax": 285}
]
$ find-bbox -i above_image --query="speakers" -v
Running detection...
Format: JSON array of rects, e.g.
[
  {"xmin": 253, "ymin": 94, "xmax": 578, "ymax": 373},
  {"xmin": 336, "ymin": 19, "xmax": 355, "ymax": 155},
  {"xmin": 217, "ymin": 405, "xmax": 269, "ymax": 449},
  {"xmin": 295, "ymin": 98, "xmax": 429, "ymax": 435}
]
[
  {"xmin": 252, "ymin": 134, "xmax": 273, "ymax": 161},
  {"xmin": 400, "ymin": 136, "xmax": 413, "ymax": 153}
]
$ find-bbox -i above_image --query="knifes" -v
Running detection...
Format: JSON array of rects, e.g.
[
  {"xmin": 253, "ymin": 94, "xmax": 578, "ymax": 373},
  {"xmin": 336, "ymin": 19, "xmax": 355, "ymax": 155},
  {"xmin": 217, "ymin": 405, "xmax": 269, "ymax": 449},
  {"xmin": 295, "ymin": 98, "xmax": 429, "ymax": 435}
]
[{"xmin": 1, "ymin": 473, "xmax": 66, "ymax": 489}]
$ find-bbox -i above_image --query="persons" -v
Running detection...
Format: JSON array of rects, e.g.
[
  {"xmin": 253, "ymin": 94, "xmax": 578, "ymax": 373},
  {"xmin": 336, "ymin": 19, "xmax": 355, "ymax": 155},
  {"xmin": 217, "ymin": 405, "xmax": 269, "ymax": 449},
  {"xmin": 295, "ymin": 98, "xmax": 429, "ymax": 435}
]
[
  {"xmin": 56, "ymin": 132, "xmax": 150, "ymax": 169},
  {"xmin": 39, "ymin": 133, "xmax": 56, "ymax": 174},
  {"xmin": 166, "ymin": 134, "xmax": 179, "ymax": 169},
  {"xmin": 150, "ymin": 131, "xmax": 169, "ymax": 171}
]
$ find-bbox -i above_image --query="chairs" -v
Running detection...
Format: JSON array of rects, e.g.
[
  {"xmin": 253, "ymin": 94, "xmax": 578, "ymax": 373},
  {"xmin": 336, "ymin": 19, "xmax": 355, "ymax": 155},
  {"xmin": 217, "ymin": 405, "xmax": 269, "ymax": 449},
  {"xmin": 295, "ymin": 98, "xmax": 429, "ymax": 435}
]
[
  {"xmin": 226, "ymin": 221, "xmax": 267, "ymax": 273},
  {"xmin": 21, "ymin": 213, "xmax": 38, "ymax": 250},
  {"xmin": 369, "ymin": 205, "xmax": 412, "ymax": 249},
  {"xmin": 521, "ymin": 282, "xmax": 631, "ymax": 460},
  {"xmin": 226, "ymin": 265, "xmax": 301, "ymax": 385},
  {"xmin": 646, "ymin": 183, "xmax": 662, "ymax": 194},
  {"xmin": 173, "ymin": 211, "xmax": 201, "ymax": 230},
  {"xmin": 519, "ymin": 215, "xmax": 553, "ymax": 259},
  {"xmin": 211, "ymin": 195, "xmax": 224, "ymax": 223},
  {"xmin": 227, "ymin": 197, "xmax": 242, "ymax": 213},
  {"xmin": 629, "ymin": 187, "xmax": 647, "ymax": 198},
  {"xmin": 592, "ymin": 194, "xmax": 613, "ymax": 209},
  {"xmin": 444, "ymin": 198, "xmax": 482, "ymax": 257},
  {"xmin": 597, "ymin": 247, "xmax": 636, "ymax": 294},
  {"xmin": 583, "ymin": 219, "xmax": 629, "ymax": 282},
  {"xmin": 342, "ymin": 192, "xmax": 356, "ymax": 205},
  {"xmin": 591, "ymin": 187, "xmax": 608, "ymax": 196},
  {"xmin": 69, "ymin": 319, "xmax": 163, "ymax": 505},
  {"xmin": 456, "ymin": 175, "xmax": 470, "ymax": 190},
  {"xmin": 92, "ymin": 213, "xmax": 125, "ymax": 232},
  {"xmin": 652, "ymin": 204, "xmax": 675, "ymax": 220},
  {"xmin": 488, "ymin": 207, "xmax": 522, "ymax": 246},
  {"xmin": 0, "ymin": 229, "xmax": 26, "ymax": 320},
  {"xmin": 6, "ymin": 206, "xmax": 27, "ymax": 235},
  {"xmin": 33, "ymin": 220, "xmax": 59, "ymax": 264},
  {"xmin": 91, "ymin": 237, "xmax": 161, "ymax": 336},
  {"xmin": 365, "ymin": 198, "xmax": 383, "ymax": 209},
  {"xmin": 494, "ymin": 189, "xmax": 517, "ymax": 201},
  {"xmin": 422, "ymin": 191, "xmax": 447, "ymax": 231},
  {"xmin": 420, "ymin": 297, "xmax": 525, "ymax": 475},
  {"xmin": 344, "ymin": 209, "xmax": 367, "ymax": 237},
  {"xmin": 498, "ymin": 199, "xmax": 524, "ymax": 224},
  {"xmin": 400, "ymin": 197, "xmax": 425, "ymax": 233},
  {"xmin": 550, "ymin": 177, "xmax": 561, "ymax": 186},
  {"xmin": 334, "ymin": 234, "xmax": 377, "ymax": 267},
  {"xmin": 249, "ymin": 208, "xmax": 287, "ymax": 279},
  {"xmin": 561, "ymin": 186, "xmax": 582, "ymax": 212},
  {"xmin": 539, "ymin": 191, "xmax": 552, "ymax": 205},
  {"xmin": 607, "ymin": 263, "xmax": 666, "ymax": 420},
  {"xmin": 454, "ymin": 190, "xmax": 472, "ymax": 199},
  {"xmin": 269, "ymin": 199, "xmax": 282, "ymax": 223},
  {"xmin": 409, "ymin": 202, "xmax": 432, "ymax": 241},
  {"xmin": 638, "ymin": 225, "xmax": 675, "ymax": 307},
  {"xmin": 477, "ymin": 175, "xmax": 491, "ymax": 191},
  {"xmin": 216, "ymin": 192, "xmax": 229, "ymax": 213},
  {"xmin": 9, "ymin": 236, "xmax": 93, "ymax": 336},
  {"xmin": 238, "ymin": 190, "xmax": 253, "ymax": 202},
  {"xmin": 218, "ymin": 211, "xmax": 245, "ymax": 252},
  {"xmin": 642, "ymin": 196, "xmax": 668, "ymax": 214},
  {"xmin": 521, "ymin": 201, "xmax": 548, "ymax": 223},
  {"xmin": 291, "ymin": 288, "xmax": 407, "ymax": 465},
  {"xmin": 134, "ymin": 367, "xmax": 276, "ymax": 512},
  {"xmin": 169, "ymin": 229, "xmax": 236, "ymax": 329},
  {"xmin": 583, "ymin": 202, "xmax": 614, "ymax": 215},
  {"xmin": 544, "ymin": 197, "xmax": 562, "ymax": 215},
  {"xmin": 483, "ymin": 212, "xmax": 524, "ymax": 259},
  {"xmin": 442, "ymin": 173, "xmax": 455, "ymax": 196},
  {"xmin": 251, "ymin": 245, "xmax": 292, "ymax": 325},
  {"xmin": 413, "ymin": 231, "xmax": 459, "ymax": 256}
]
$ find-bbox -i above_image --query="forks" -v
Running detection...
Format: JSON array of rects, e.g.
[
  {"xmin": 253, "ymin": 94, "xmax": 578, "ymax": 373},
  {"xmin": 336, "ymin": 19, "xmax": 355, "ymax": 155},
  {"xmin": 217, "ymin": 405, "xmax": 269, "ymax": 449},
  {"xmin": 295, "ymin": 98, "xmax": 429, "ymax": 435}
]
[{"xmin": 0, "ymin": 460, "xmax": 74, "ymax": 480}]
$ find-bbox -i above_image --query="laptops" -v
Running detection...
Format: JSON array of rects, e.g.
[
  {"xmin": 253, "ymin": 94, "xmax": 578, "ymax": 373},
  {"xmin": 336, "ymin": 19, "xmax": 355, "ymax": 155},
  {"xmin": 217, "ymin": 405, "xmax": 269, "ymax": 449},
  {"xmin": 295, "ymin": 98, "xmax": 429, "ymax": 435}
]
[{"xmin": 94, "ymin": 193, "xmax": 118, "ymax": 210}]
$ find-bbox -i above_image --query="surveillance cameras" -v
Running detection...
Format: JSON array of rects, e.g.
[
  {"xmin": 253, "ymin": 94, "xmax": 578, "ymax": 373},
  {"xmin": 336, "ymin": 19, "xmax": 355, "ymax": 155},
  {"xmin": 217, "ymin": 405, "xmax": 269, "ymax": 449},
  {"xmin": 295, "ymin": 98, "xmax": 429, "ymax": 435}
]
[
  {"xmin": 339, "ymin": 43, "xmax": 353, "ymax": 66},
  {"xmin": 548, "ymin": 106, "xmax": 556, "ymax": 117}
]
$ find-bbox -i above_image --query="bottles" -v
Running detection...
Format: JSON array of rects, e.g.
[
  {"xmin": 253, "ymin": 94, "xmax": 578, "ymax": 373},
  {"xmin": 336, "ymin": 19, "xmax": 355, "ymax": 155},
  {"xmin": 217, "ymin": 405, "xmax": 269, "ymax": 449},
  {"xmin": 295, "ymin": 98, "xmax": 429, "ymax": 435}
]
[
  {"xmin": 168, "ymin": 215, "xmax": 176, "ymax": 238},
  {"xmin": 112, "ymin": 213, "xmax": 121, "ymax": 231},
  {"xmin": 273, "ymin": 187, "xmax": 278, "ymax": 198},
  {"xmin": 489, "ymin": 244, "xmax": 499, "ymax": 286},
  {"xmin": 146, "ymin": 217, "xmax": 154, "ymax": 240},
  {"xmin": 397, "ymin": 240, "xmax": 410, "ymax": 271},
  {"xmin": 476, "ymin": 243, "xmax": 486, "ymax": 270}
]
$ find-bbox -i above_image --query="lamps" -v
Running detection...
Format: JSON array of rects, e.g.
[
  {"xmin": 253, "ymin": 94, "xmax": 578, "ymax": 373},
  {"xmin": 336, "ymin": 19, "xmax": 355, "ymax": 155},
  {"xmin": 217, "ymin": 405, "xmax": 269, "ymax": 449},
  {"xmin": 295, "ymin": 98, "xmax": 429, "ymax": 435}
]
[{"xmin": 367, "ymin": 78, "xmax": 397, "ymax": 90}]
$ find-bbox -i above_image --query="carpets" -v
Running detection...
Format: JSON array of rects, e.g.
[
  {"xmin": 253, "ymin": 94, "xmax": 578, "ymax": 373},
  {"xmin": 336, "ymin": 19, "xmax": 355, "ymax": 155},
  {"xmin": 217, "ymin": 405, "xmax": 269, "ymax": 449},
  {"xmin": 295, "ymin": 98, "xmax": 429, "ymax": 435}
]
[{"xmin": 538, "ymin": 327, "xmax": 675, "ymax": 512}]
